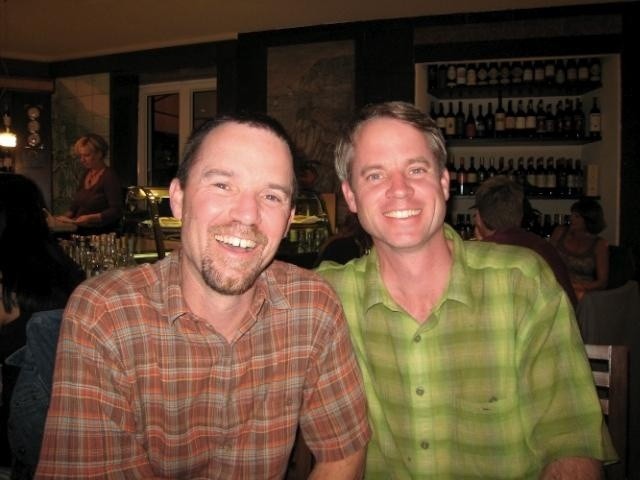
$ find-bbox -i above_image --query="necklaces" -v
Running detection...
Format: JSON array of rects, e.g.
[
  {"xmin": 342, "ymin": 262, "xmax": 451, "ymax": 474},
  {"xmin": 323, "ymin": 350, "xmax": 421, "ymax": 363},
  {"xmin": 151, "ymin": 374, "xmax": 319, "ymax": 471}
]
[{"xmin": 88, "ymin": 167, "xmax": 105, "ymax": 183}]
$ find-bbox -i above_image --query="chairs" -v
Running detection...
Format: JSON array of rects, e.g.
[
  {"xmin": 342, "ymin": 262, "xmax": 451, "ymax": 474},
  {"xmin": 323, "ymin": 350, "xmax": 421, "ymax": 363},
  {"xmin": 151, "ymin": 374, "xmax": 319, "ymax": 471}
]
[{"xmin": 578, "ymin": 237, "xmax": 640, "ymax": 480}]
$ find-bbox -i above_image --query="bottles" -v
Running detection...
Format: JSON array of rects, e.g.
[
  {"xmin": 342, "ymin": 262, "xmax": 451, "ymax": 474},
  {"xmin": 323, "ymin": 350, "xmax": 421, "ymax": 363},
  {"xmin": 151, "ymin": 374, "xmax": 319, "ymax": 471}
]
[
  {"xmin": 60, "ymin": 231, "xmax": 138, "ymax": 278},
  {"xmin": 290, "ymin": 228, "xmax": 323, "ymax": 255},
  {"xmin": 426, "ymin": 59, "xmax": 603, "ymax": 198},
  {"xmin": 454, "ymin": 212, "xmax": 472, "ymax": 240},
  {"xmin": 533, "ymin": 211, "xmax": 571, "ymax": 240}
]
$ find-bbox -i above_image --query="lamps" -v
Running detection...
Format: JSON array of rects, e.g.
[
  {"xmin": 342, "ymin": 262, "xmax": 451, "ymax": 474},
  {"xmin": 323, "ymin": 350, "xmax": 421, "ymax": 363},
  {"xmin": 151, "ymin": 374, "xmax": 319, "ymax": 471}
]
[{"xmin": 0, "ymin": 90, "xmax": 18, "ymax": 148}]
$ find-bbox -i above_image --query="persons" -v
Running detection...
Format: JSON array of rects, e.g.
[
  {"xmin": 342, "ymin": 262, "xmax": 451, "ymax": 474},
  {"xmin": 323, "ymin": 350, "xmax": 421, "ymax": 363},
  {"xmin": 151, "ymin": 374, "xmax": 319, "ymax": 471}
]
[
  {"xmin": 475, "ymin": 175, "xmax": 577, "ymax": 310},
  {"xmin": 55, "ymin": 134, "xmax": 122, "ymax": 238},
  {"xmin": 32, "ymin": 107, "xmax": 371, "ymax": 480},
  {"xmin": 310, "ymin": 101, "xmax": 620, "ymax": 480},
  {"xmin": 0, "ymin": 173, "xmax": 85, "ymax": 466},
  {"xmin": 550, "ymin": 199, "xmax": 610, "ymax": 303}
]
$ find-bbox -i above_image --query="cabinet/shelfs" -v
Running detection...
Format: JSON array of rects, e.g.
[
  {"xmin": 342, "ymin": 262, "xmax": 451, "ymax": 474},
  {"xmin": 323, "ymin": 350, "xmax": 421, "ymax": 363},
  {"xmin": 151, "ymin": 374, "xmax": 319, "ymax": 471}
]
[
  {"xmin": 115, "ymin": 185, "xmax": 333, "ymax": 264},
  {"xmin": 8, "ymin": 105, "xmax": 53, "ymax": 218},
  {"xmin": 411, "ymin": 54, "xmax": 621, "ymax": 247}
]
